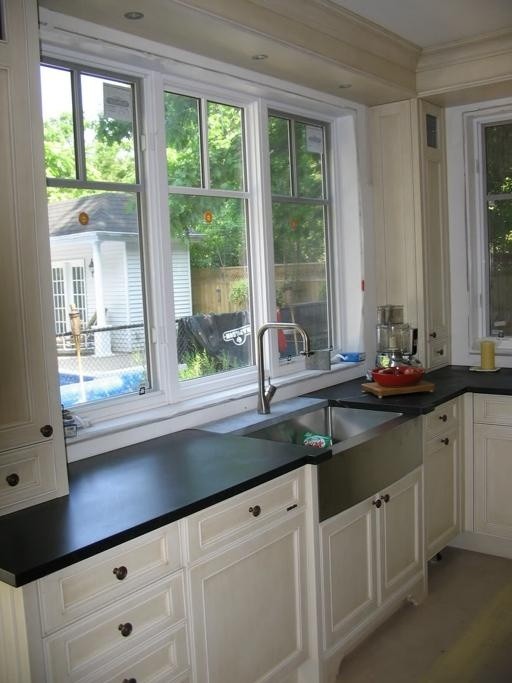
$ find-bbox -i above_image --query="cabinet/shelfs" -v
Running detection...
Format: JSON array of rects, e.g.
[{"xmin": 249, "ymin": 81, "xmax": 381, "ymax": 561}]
[
  {"xmin": 317, "ymin": 463, "xmax": 426, "ymax": 682},
  {"xmin": 367, "ymin": 100, "xmax": 452, "ymax": 373},
  {"xmin": 0, "ymin": 462, "xmax": 316, "ymax": 682},
  {"xmin": 0, "ymin": 40, "xmax": 71, "ymax": 518},
  {"xmin": 422, "ymin": 393, "xmax": 465, "ymax": 564},
  {"xmin": 466, "ymin": 391, "xmax": 511, "ymax": 560}
]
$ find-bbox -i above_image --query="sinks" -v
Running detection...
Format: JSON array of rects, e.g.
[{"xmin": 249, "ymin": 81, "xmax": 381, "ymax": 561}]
[{"xmin": 242, "ymin": 403, "xmax": 423, "ymax": 523}]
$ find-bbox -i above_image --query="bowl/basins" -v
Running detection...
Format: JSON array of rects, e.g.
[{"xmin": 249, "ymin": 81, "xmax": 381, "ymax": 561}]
[{"xmin": 370, "ymin": 364, "xmax": 425, "ymax": 387}]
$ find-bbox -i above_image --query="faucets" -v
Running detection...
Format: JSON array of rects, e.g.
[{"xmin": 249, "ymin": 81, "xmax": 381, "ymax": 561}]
[{"xmin": 256, "ymin": 322, "xmax": 314, "ymax": 413}]
